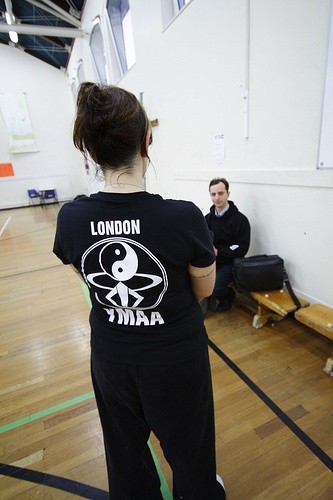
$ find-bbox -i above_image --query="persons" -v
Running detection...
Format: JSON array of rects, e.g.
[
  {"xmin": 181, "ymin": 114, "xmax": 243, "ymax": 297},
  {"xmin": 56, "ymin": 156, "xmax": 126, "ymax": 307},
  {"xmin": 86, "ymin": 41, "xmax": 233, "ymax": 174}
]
[
  {"xmin": 205, "ymin": 177, "xmax": 251, "ymax": 313},
  {"xmin": 52, "ymin": 80, "xmax": 226, "ymax": 500}
]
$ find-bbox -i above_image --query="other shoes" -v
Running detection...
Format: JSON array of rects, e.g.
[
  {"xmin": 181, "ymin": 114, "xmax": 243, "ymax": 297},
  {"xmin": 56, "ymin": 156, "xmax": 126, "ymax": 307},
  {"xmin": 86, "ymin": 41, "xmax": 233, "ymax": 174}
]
[
  {"xmin": 216, "ymin": 287, "xmax": 236, "ymax": 312},
  {"xmin": 216, "ymin": 474, "xmax": 225, "ymax": 490}
]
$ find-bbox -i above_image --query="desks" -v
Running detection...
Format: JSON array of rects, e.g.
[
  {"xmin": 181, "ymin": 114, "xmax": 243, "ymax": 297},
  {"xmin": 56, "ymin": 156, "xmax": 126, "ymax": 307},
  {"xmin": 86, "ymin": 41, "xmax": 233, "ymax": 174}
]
[{"xmin": 38, "ymin": 189, "xmax": 59, "ymax": 208}]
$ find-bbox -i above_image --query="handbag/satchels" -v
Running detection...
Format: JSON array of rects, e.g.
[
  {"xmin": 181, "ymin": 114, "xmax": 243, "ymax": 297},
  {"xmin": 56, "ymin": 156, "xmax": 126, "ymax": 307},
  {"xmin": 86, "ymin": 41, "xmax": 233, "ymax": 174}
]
[{"xmin": 231, "ymin": 255, "xmax": 289, "ymax": 291}]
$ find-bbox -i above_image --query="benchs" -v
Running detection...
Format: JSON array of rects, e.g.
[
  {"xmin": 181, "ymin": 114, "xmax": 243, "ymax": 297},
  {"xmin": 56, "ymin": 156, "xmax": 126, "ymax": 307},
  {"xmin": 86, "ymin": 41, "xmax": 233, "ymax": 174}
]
[{"xmin": 249, "ymin": 289, "xmax": 333, "ymax": 379}]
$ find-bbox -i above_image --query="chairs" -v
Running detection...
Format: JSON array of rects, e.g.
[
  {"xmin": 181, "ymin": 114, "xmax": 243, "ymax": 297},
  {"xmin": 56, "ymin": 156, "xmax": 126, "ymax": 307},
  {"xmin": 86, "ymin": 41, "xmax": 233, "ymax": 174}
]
[{"xmin": 26, "ymin": 188, "xmax": 61, "ymax": 211}]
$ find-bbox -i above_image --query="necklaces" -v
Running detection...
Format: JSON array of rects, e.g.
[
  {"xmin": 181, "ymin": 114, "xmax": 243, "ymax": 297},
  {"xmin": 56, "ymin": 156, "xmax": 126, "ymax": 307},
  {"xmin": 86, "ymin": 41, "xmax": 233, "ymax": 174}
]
[{"xmin": 104, "ymin": 183, "xmax": 145, "ymax": 191}]
